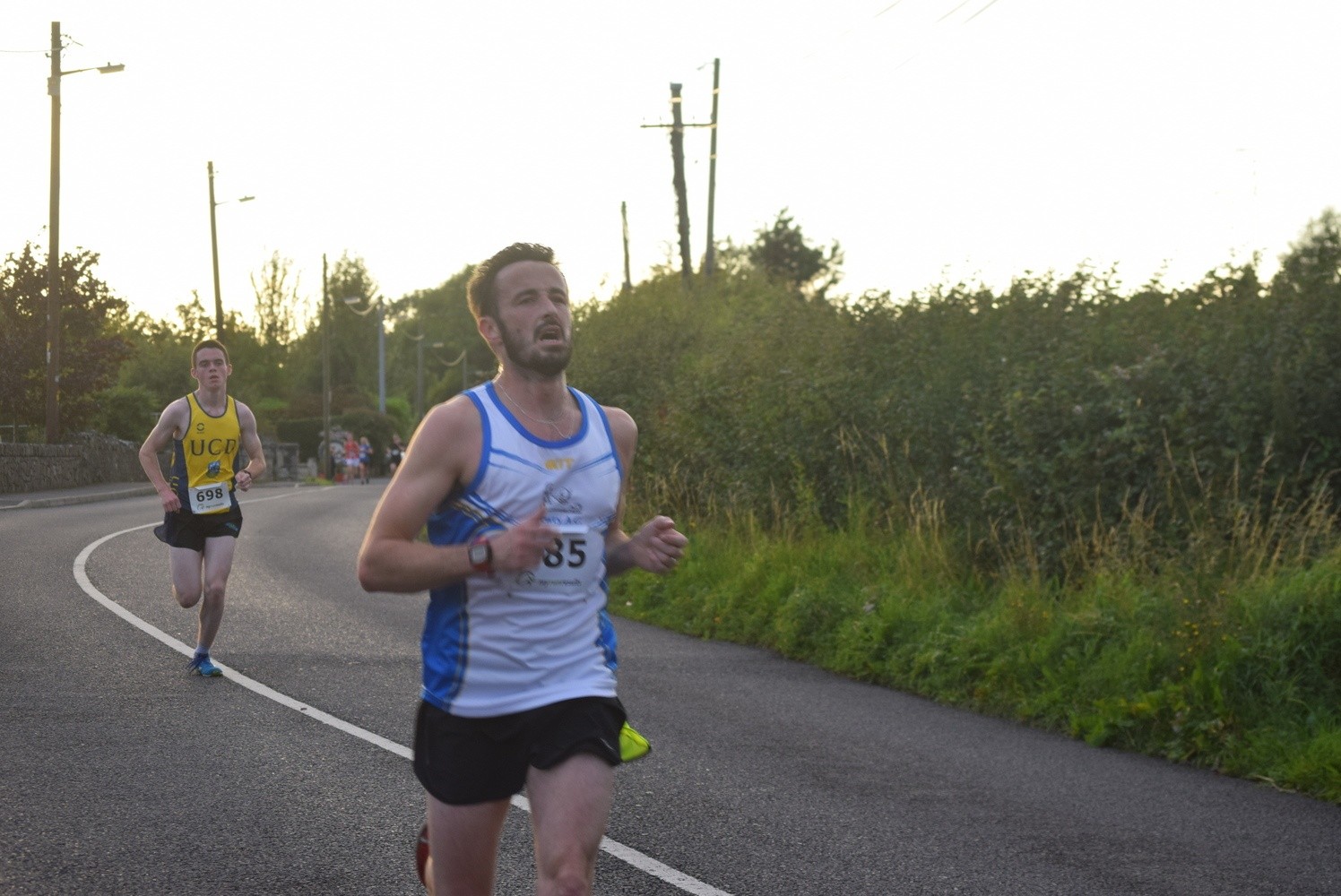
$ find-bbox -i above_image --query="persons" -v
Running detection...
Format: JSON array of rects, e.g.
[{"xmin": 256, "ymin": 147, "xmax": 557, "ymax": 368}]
[
  {"xmin": 331, "ymin": 430, "xmax": 407, "ymax": 485},
  {"xmin": 359, "ymin": 242, "xmax": 690, "ymax": 895},
  {"xmin": 139, "ymin": 340, "xmax": 267, "ymax": 677}
]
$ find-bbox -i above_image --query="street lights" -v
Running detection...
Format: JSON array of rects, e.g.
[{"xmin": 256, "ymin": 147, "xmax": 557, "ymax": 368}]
[
  {"xmin": 44, "ymin": 63, "xmax": 126, "ymax": 444},
  {"xmin": 210, "ymin": 195, "xmax": 256, "ymax": 343},
  {"xmin": 320, "ymin": 295, "xmax": 360, "ymax": 481}
]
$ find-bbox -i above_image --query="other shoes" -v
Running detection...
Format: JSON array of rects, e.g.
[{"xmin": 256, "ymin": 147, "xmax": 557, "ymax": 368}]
[{"xmin": 415, "ymin": 823, "xmax": 433, "ymax": 889}]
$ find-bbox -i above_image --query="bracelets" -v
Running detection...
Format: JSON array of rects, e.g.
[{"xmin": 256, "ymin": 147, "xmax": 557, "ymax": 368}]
[{"xmin": 242, "ymin": 469, "xmax": 251, "ymax": 478}]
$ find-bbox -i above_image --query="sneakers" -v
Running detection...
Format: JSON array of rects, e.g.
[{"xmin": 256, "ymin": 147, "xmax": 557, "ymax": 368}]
[{"xmin": 185, "ymin": 651, "xmax": 222, "ymax": 677}]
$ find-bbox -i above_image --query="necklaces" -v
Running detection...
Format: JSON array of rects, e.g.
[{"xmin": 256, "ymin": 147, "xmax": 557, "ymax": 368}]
[{"xmin": 493, "ymin": 377, "xmax": 574, "ymax": 440}]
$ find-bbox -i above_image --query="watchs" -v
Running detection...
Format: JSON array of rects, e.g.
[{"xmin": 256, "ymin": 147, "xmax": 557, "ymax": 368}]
[{"xmin": 468, "ymin": 536, "xmax": 496, "ymax": 579}]
